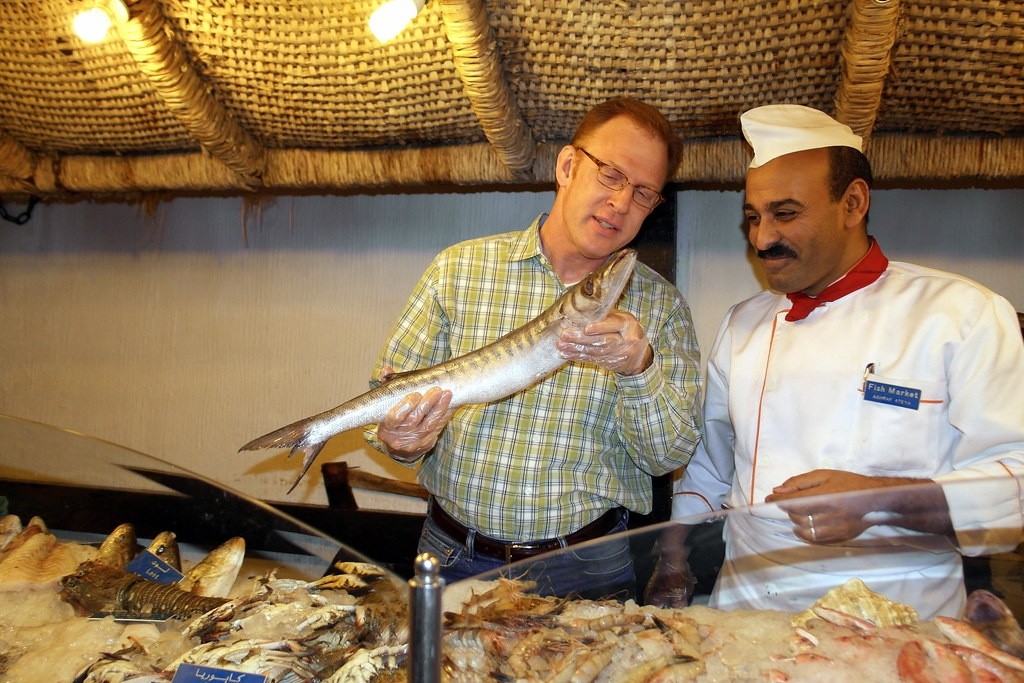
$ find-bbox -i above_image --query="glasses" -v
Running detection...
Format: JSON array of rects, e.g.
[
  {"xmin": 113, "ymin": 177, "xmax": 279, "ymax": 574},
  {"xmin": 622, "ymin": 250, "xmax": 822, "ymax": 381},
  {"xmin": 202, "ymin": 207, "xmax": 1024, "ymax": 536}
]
[{"xmin": 574, "ymin": 146, "xmax": 664, "ymax": 213}]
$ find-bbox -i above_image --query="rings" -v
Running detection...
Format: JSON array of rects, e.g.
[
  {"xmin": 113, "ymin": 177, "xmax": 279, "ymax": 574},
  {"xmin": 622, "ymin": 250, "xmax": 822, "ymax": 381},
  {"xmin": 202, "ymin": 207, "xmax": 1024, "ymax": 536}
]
[
  {"xmin": 810, "ymin": 528, "xmax": 816, "ymax": 540},
  {"xmin": 808, "ymin": 515, "xmax": 813, "ymax": 527}
]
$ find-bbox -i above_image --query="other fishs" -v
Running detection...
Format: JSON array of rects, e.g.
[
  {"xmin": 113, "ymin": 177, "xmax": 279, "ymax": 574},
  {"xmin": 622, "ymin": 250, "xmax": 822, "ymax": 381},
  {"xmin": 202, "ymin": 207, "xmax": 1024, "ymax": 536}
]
[
  {"xmin": 237, "ymin": 247, "xmax": 638, "ymax": 496},
  {"xmin": 0, "ymin": 514, "xmax": 245, "ymax": 683}
]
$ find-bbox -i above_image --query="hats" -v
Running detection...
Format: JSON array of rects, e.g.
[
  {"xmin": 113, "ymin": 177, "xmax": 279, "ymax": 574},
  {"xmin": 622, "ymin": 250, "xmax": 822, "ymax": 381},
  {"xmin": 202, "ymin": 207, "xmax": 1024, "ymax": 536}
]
[{"xmin": 740, "ymin": 104, "xmax": 864, "ymax": 169}]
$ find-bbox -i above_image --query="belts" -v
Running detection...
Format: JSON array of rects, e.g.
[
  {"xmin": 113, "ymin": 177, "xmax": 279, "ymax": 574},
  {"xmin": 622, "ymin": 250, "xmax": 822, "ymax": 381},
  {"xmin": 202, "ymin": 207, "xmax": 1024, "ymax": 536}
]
[{"xmin": 426, "ymin": 495, "xmax": 628, "ymax": 564}]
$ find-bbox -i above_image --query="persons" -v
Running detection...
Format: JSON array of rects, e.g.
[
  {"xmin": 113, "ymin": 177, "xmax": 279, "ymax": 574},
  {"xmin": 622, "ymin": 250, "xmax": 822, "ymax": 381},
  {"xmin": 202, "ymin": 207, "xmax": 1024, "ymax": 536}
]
[
  {"xmin": 642, "ymin": 103, "xmax": 1024, "ymax": 619},
  {"xmin": 364, "ymin": 99, "xmax": 705, "ymax": 601}
]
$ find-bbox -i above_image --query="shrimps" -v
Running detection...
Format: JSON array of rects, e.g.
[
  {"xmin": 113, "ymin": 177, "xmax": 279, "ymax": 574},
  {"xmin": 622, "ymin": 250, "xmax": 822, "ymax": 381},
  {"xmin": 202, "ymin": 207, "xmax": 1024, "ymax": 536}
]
[
  {"xmin": 58, "ymin": 559, "xmax": 232, "ymax": 622},
  {"xmin": 441, "ymin": 576, "xmax": 1024, "ymax": 683}
]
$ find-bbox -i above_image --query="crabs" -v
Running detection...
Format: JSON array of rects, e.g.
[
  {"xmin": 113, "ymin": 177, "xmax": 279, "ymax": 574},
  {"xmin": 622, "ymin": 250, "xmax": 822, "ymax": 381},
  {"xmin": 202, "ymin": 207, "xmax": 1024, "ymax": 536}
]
[{"xmin": 73, "ymin": 561, "xmax": 411, "ymax": 683}]
